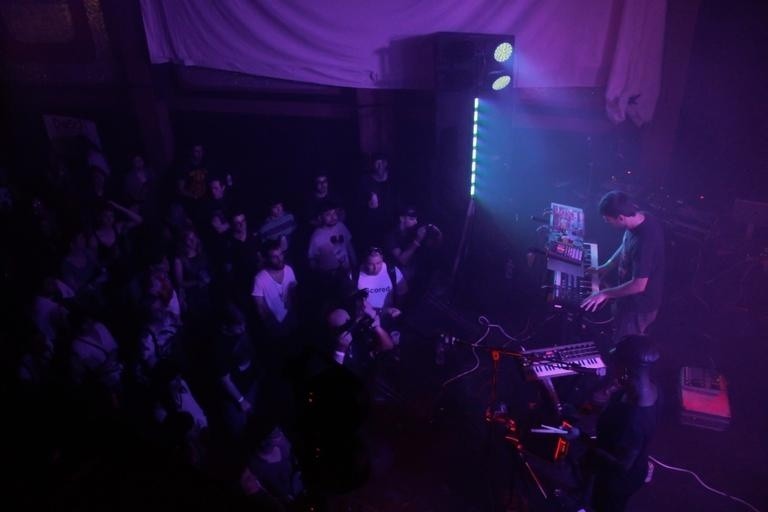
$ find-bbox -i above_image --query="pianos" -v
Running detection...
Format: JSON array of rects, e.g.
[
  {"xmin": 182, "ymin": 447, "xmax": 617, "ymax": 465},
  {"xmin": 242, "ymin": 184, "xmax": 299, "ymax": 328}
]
[
  {"xmin": 553, "ymin": 241, "xmax": 599, "ymax": 311},
  {"xmin": 519, "ymin": 342, "xmax": 606, "ymax": 381}
]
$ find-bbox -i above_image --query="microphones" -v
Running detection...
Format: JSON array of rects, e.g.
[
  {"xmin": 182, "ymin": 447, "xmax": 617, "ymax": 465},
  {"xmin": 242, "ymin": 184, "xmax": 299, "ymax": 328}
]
[{"xmin": 570, "ymin": 367, "xmax": 607, "ymax": 376}]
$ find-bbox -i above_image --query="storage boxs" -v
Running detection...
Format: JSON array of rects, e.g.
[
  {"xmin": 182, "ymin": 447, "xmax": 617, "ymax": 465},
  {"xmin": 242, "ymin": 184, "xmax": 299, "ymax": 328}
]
[{"xmin": 678, "ymin": 365, "xmax": 731, "ymax": 433}]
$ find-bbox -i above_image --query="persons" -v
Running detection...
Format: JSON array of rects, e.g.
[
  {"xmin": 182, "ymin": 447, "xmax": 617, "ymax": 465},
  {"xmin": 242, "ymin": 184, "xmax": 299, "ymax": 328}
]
[
  {"xmin": 1, "ymin": 143, "xmax": 444, "ymax": 510},
  {"xmin": 578, "ymin": 190, "xmax": 663, "ymax": 403}
]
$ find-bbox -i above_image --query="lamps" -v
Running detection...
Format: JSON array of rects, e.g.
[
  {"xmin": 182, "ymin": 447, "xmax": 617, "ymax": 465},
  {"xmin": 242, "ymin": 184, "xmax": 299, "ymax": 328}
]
[{"xmin": 484, "ymin": 34, "xmax": 515, "ymax": 99}]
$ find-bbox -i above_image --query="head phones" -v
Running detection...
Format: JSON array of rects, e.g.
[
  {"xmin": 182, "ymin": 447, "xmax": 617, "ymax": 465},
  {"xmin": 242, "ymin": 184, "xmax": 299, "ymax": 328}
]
[{"xmin": 617, "ymin": 363, "xmax": 634, "ymax": 387}]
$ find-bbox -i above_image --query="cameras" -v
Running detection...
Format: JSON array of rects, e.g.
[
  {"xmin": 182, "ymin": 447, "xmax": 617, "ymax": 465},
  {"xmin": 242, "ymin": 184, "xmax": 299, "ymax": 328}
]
[
  {"xmin": 342, "ymin": 315, "xmax": 376, "ymax": 344},
  {"xmin": 426, "ymin": 223, "xmax": 440, "ymax": 241}
]
